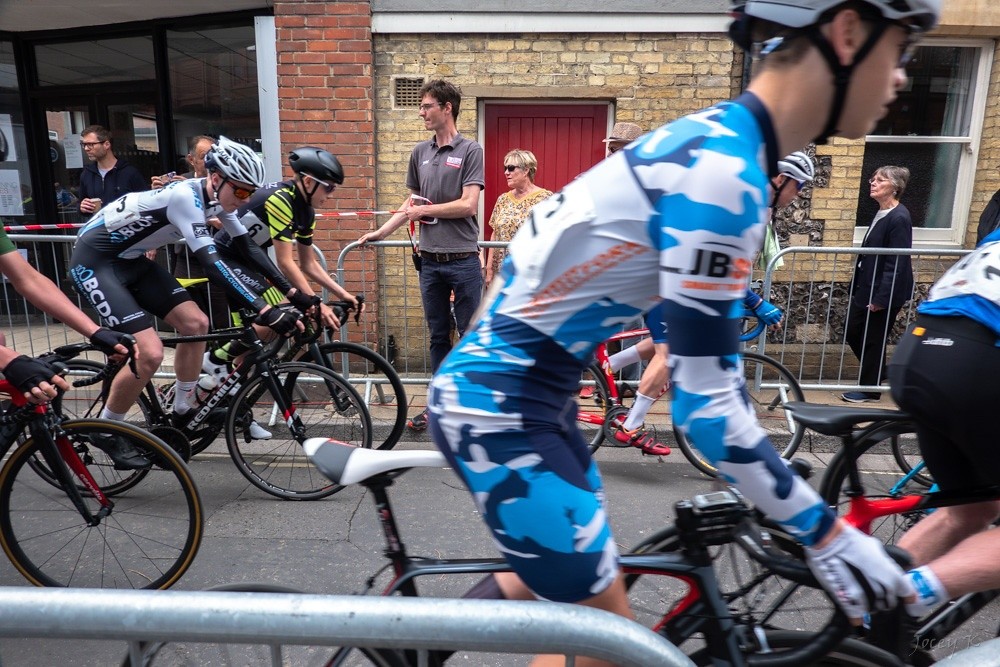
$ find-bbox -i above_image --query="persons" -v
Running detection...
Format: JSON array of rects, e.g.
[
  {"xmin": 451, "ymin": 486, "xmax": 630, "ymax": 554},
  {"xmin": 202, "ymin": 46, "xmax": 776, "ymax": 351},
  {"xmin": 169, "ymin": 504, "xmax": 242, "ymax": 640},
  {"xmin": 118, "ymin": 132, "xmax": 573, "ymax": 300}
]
[
  {"xmin": 0, "ymin": 220, "xmax": 140, "ymax": 404},
  {"xmin": 608, "ymin": 152, "xmax": 815, "ymax": 455},
  {"xmin": 579, "ymin": 121, "xmax": 647, "ymax": 399},
  {"xmin": 484, "ymin": 148, "xmax": 554, "ymax": 288},
  {"xmin": 884, "ymin": 227, "xmax": 1000, "ymax": 625},
  {"xmin": 68, "ymin": 123, "xmax": 365, "ymax": 468},
  {"xmin": 357, "ymin": 79, "xmax": 485, "ymax": 433},
  {"xmin": 841, "ymin": 166, "xmax": 913, "ymax": 403},
  {"xmin": 427, "ymin": 0, "xmax": 913, "ymax": 667}
]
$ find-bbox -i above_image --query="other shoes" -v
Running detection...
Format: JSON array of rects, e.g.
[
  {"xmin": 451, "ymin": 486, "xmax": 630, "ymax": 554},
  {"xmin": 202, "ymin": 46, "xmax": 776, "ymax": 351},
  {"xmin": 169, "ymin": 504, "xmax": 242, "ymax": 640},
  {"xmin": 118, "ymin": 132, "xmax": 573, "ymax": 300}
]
[
  {"xmin": 88, "ymin": 429, "xmax": 151, "ymax": 471},
  {"xmin": 171, "ymin": 405, "xmax": 229, "ymax": 429},
  {"xmin": 841, "ymin": 391, "xmax": 881, "ymax": 404}
]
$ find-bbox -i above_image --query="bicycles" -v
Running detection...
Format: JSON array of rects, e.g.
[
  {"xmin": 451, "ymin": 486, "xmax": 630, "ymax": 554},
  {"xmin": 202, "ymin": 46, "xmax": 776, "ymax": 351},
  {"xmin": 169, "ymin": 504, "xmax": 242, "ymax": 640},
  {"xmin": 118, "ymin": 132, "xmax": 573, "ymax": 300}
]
[
  {"xmin": 9, "ymin": 301, "xmax": 373, "ymax": 502},
  {"xmin": 630, "ymin": 398, "xmax": 1000, "ymax": 667},
  {"xmin": 570, "ymin": 300, "xmax": 805, "ymax": 482},
  {"xmin": 0, "ymin": 338, "xmax": 207, "ymax": 592},
  {"xmin": 101, "ymin": 275, "xmax": 408, "ymax": 458},
  {"xmin": 119, "ymin": 439, "xmax": 910, "ymax": 667}
]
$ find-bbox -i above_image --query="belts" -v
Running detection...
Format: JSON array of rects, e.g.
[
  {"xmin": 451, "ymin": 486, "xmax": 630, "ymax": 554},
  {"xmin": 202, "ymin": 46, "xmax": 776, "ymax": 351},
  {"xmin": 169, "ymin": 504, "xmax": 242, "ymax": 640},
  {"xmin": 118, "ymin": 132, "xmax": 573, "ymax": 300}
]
[{"xmin": 420, "ymin": 250, "xmax": 479, "ymax": 263}]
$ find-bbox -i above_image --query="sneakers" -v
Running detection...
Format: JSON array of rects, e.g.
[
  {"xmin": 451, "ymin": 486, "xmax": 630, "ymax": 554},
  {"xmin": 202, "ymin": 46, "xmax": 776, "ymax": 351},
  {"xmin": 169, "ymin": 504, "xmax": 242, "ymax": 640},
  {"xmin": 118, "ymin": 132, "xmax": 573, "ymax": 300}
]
[
  {"xmin": 615, "ymin": 422, "xmax": 671, "ymax": 455},
  {"xmin": 250, "ymin": 420, "xmax": 273, "ymax": 440},
  {"xmin": 406, "ymin": 409, "xmax": 430, "ymax": 431},
  {"xmin": 202, "ymin": 352, "xmax": 229, "ymax": 383}
]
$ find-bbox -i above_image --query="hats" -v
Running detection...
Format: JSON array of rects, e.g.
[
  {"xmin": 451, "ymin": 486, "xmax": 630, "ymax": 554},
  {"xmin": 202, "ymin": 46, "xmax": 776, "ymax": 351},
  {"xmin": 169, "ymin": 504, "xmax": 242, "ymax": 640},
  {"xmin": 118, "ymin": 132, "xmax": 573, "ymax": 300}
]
[{"xmin": 602, "ymin": 122, "xmax": 643, "ymax": 142}]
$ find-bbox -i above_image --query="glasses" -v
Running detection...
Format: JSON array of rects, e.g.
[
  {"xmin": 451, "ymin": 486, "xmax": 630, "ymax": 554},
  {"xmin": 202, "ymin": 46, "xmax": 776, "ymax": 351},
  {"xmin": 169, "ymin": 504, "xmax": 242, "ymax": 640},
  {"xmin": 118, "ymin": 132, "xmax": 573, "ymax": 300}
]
[
  {"xmin": 795, "ymin": 181, "xmax": 806, "ymax": 191},
  {"xmin": 607, "ymin": 146, "xmax": 616, "ymax": 152},
  {"xmin": 897, "ymin": 38, "xmax": 920, "ymax": 69},
  {"xmin": 504, "ymin": 164, "xmax": 520, "ymax": 171},
  {"xmin": 419, "ymin": 102, "xmax": 441, "ymax": 111},
  {"xmin": 79, "ymin": 141, "xmax": 104, "ymax": 149},
  {"xmin": 219, "ymin": 175, "xmax": 258, "ymax": 200},
  {"xmin": 325, "ymin": 184, "xmax": 336, "ymax": 194},
  {"xmin": 868, "ymin": 178, "xmax": 888, "ymax": 183}
]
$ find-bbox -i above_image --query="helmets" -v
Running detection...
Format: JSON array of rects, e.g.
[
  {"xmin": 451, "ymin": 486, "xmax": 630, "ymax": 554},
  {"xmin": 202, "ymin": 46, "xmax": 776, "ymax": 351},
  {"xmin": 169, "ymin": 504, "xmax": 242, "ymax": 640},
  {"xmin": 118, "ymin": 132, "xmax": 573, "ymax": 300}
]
[
  {"xmin": 203, "ymin": 135, "xmax": 266, "ymax": 189},
  {"xmin": 744, "ymin": 0, "xmax": 939, "ymax": 37},
  {"xmin": 777, "ymin": 152, "xmax": 815, "ymax": 182},
  {"xmin": 288, "ymin": 146, "xmax": 344, "ymax": 186}
]
